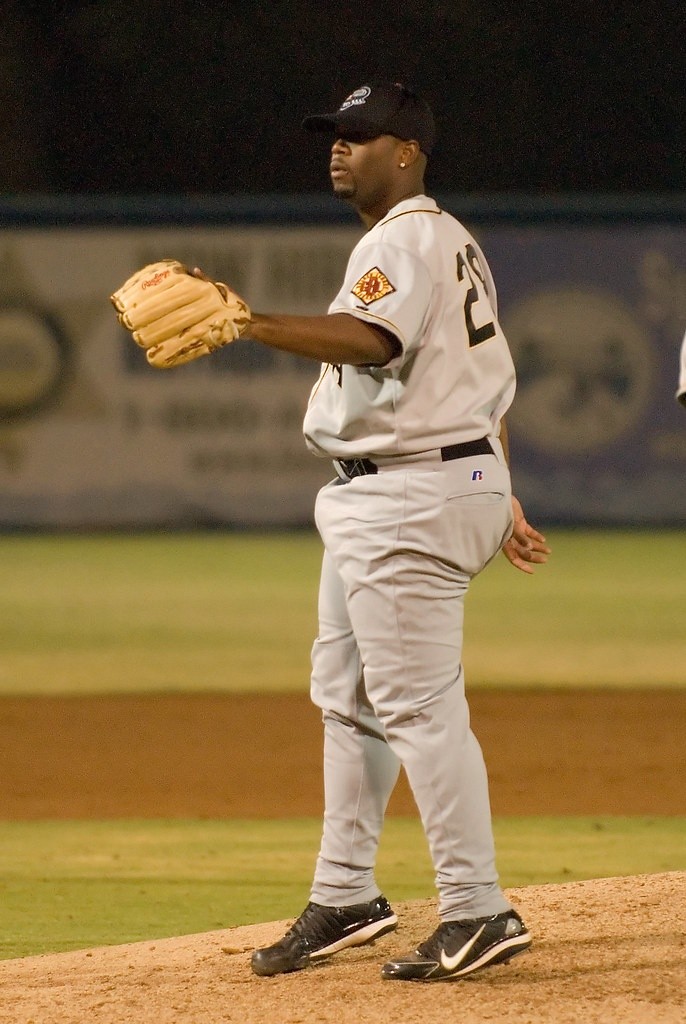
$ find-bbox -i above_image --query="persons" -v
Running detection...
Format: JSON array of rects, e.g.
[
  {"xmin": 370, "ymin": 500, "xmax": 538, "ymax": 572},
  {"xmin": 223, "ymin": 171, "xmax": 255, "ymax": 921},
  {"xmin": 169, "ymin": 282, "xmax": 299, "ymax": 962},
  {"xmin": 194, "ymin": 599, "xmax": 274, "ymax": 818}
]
[{"xmin": 108, "ymin": 84, "xmax": 550, "ymax": 985}]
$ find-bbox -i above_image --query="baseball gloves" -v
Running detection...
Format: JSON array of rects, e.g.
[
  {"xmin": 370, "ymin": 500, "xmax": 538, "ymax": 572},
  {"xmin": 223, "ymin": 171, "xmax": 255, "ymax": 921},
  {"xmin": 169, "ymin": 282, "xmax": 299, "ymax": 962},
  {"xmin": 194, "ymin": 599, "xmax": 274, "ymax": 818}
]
[{"xmin": 110, "ymin": 260, "xmax": 254, "ymax": 371}]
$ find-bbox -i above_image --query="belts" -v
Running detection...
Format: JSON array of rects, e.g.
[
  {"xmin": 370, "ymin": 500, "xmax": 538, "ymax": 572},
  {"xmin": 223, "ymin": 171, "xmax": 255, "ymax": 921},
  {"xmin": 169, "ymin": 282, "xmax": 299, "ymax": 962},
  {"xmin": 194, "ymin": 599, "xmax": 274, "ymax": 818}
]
[{"xmin": 332, "ymin": 437, "xmax": 495, "ymax": 480}]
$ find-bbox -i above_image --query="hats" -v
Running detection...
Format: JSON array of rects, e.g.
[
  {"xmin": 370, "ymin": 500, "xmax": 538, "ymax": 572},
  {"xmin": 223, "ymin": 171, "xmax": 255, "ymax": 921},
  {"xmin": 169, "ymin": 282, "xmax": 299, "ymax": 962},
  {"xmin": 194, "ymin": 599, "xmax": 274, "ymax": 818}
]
[{"xmin": 300, "ymin": 79, "xmax": 436, "ymax": 155}]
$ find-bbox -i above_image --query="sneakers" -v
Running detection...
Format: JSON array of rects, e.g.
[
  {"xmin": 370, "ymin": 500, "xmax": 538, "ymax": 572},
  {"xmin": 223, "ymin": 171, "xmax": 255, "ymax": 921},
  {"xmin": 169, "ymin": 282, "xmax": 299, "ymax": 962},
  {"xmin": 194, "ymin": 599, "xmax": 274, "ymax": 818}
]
[
  {"xmin": 250, "ymin": 891, "xmax": 398, "ymax": 976},
  {"xmin": 380, "ymin": 906, "xmax": 533, "ymax": 981}
]
